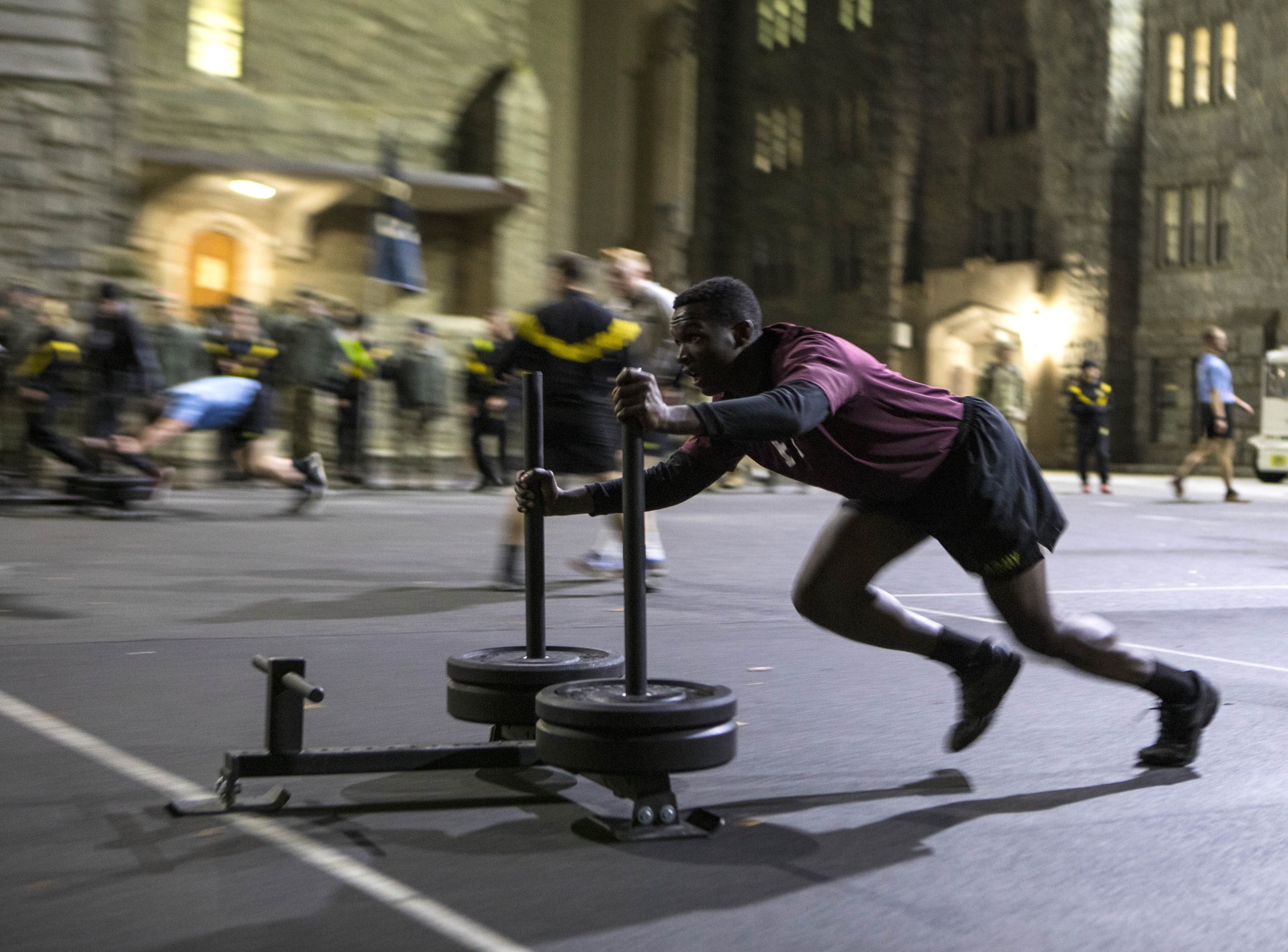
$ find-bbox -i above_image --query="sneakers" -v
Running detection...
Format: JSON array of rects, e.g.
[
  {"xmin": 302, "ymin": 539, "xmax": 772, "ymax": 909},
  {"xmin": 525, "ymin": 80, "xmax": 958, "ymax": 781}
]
[
  {"xmin": 1138, "ymin": 669, "xmax": 1221, "ymax": 768},
  {"xmin": 582, "ymin": 545, "xmax": 672, "ymax": 579},
  {"xmin": 949, "ymin": 639, "xmax": 1023, "ymax": 751},
  {"xmin": 491, "ymin": 570, "xmax": 542, "ymax": 591},
  {"xmin": 295, "ymin": 453, "xmax": 328, "ymax": 517}
]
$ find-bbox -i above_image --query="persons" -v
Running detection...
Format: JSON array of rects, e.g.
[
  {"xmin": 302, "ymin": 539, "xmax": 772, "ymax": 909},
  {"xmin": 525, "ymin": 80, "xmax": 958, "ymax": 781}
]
[
  {"xmin": 515, "ymin": 275, "xmax": 1220, "ymax": 768},
  {"xmin": 0, "ymin": 247, "xmax": 772, "ymax": 592},
  {"xmin": 1170, "ymin": 323, "xmax": 1256, "ymax": 505},
  {"xmin": 1061, "ymin": 359, "xmax": 1117, "ymax": 497}
]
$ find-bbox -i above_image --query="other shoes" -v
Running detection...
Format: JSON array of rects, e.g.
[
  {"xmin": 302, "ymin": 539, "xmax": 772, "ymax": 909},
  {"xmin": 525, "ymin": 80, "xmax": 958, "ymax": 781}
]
[
  {"xmin": 1225, "ymin": 494, "xmax": 1251, "ymax": 504},
  {"xmin": 1171, "ymin": 480, "xmax": 1184, "ymax": 495},
  {"xmin": 1082, "ymin": 483, "xmax": 1111, "ymax": 494}
]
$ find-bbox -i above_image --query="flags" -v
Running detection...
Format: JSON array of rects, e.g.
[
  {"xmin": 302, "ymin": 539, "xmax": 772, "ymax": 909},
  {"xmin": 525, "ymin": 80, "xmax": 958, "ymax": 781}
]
[{"xmin": 367, "ymin": 140, "xmax": 430, "ymax": 300}]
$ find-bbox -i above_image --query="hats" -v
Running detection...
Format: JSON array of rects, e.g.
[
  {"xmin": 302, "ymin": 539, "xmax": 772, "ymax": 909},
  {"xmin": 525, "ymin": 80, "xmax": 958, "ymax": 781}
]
[
  {"xmin": 604, "ymin": 244, "xmax": 653, "ymax": 272},
  {"xmin": 1082, "ymin": 359, "xmax": 1097, "ymax": 369}
]
